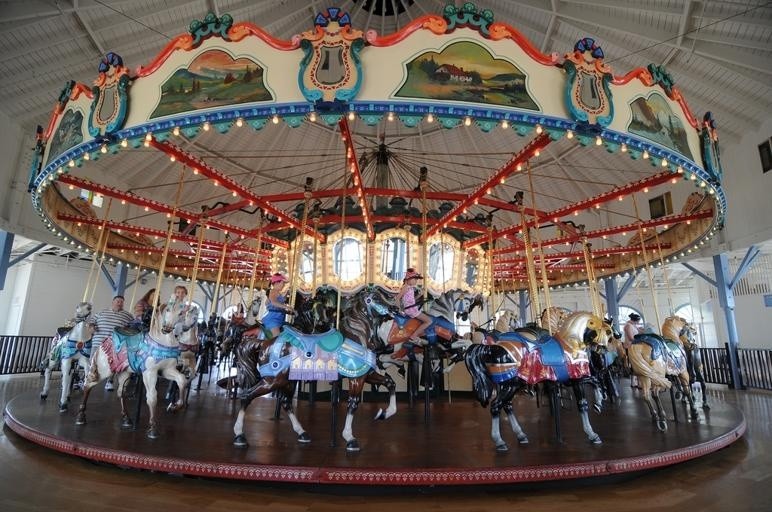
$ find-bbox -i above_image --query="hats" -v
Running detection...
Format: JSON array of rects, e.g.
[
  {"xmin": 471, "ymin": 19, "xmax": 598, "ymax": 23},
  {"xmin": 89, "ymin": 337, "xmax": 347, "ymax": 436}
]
[
  {"xmin": 272, "ymin": 273, "xmax": 287, "ymax": 283},
  {"xmin": 406, "ymin": 269, "xmax": 422, "ymax": 279}
]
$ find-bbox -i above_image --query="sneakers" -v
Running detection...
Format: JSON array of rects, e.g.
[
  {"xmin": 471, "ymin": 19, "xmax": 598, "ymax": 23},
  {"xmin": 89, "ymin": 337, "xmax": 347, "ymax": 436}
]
[
  {"xmin": 104, "ymin": 379, "xmax": 114, "ymax": 390},
  {"xmin": 409, "ymin": 334, "xmax": 422, "ymax": 343}
]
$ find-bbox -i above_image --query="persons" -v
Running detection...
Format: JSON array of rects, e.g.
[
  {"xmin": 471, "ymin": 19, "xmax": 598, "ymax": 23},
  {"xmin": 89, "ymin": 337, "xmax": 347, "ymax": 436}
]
[
  {"xmin": 624, "ymin": 313, "xmax": 641, "ymax": 388},
  {"xmin": 261, "ymin": 272, "xmax": 292, "ymax": 339},
  {"xmin": 396, "ymin": 269, "xmax": 433, "ymax": 346},
  {"xmin": 87, "ymin": 286, "xmax": 188, "ymax": 363},
  {"xmin": 231, "ymin": 303, "xmax": 246, "ymax": 325}
]
